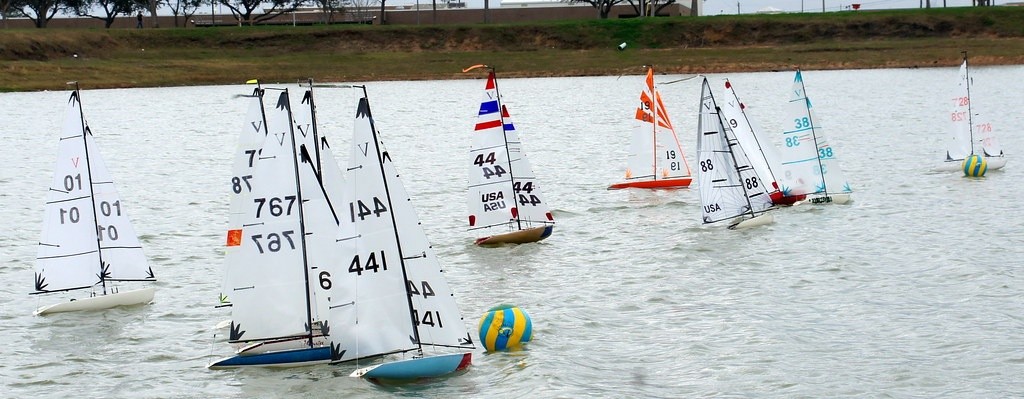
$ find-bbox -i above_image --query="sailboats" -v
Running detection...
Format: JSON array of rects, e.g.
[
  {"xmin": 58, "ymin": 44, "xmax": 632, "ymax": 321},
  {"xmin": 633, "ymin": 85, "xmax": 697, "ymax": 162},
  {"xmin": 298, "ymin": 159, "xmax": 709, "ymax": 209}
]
[
  {"xmin": 779, "ymin": 65, "xmax": 854, "ymax": 207},
  {"xmin": 605, "ymin": 63, "xmax": 694, "ymax": 190},
  {"xmin": 692, "ymin": 74, "xmax": 789, "ymax": 232},
  {"xmin": 455, "ymin": 64, "xmax": 556, "ymax": 246},
  {"xmin": 306, "ymin": 84, "xmax": 480, "ymax": 381},
  {"xmin": 926, "ymin": 50, "xmax": 1007, "ymax": 173},
  {"xmin": 203, "ymin": 73, "xmax": 387, "ymax": 370},
  {"xmin": 719, "ymin": 76, "xmax": 808, "ymax": 209},
  {"xmin": 25, "ymin": 80, "xmax": 158, "ymax": 316}
]
